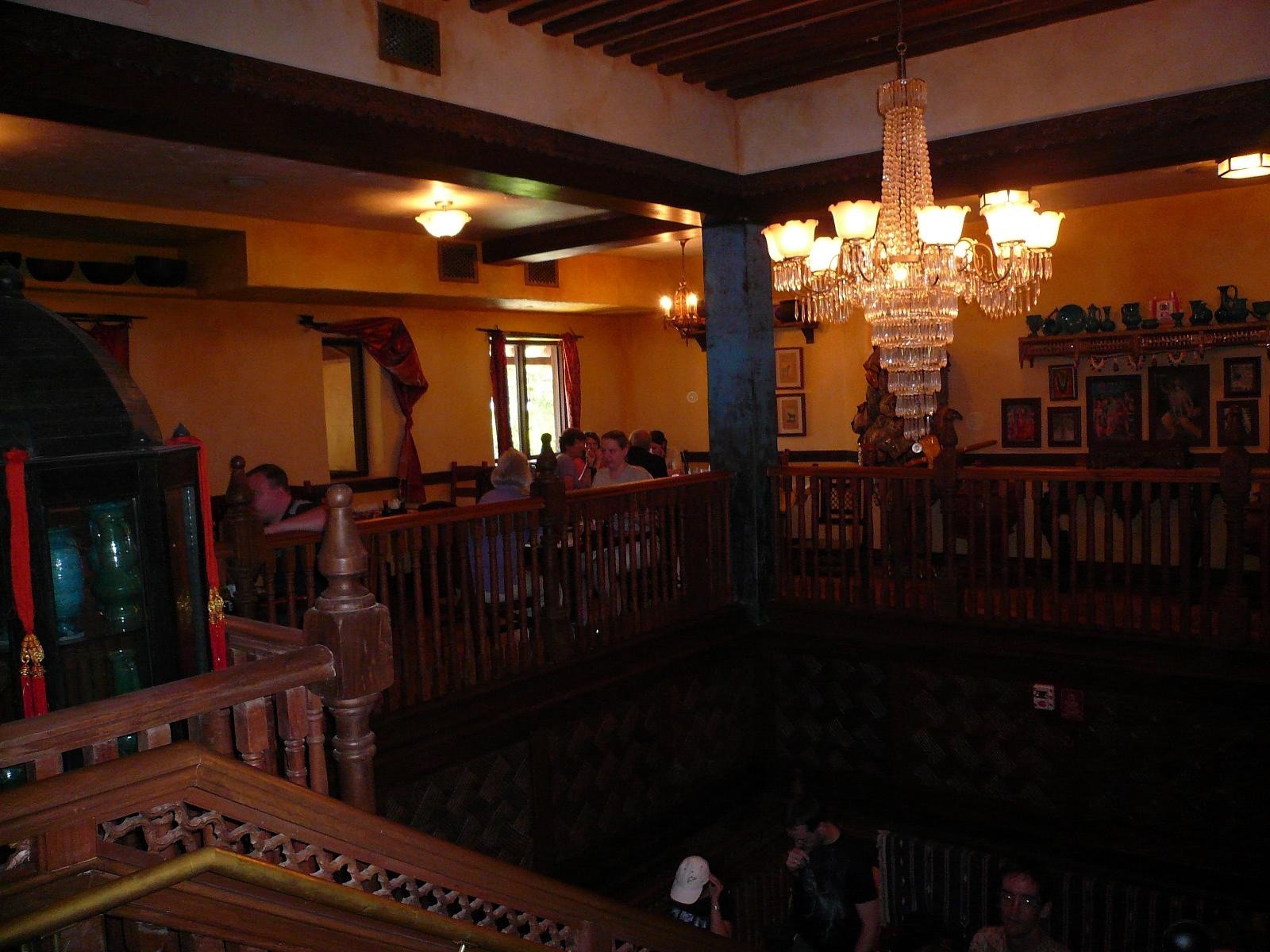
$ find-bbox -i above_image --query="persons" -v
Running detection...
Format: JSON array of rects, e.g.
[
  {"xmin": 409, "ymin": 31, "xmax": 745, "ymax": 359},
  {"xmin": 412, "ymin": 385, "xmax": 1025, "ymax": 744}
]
[
  {"xmin": 245, "ymin": 464, "xmax": 329, "ymax": 630},
  {"xmin": 969, "ymin": 868, "xmax": 1064, "ymax": 951},
  {"xmin": 556, "ymin": 428, "xmax": 682, "ymax": 628},
  {"xmin": 669, "ymin": 856, "xmax": 731, "ymax": 939},
  {"xmin": 468, "ymin": 448, "xmax": 539, "ymax": 616},
  {"xmin": 785, "ymin": 811, "xmax": 881, "ymax": 952}
]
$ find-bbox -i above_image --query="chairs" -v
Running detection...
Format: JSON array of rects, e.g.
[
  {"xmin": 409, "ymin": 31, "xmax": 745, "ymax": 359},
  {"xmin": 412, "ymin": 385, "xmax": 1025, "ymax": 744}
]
[{"xmin": 303, "ymin": 451, "xmax": 712, "ymax": 663}]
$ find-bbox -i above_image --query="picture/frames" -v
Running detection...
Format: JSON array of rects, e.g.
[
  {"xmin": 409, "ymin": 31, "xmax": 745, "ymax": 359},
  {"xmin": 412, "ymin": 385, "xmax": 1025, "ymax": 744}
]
[
  {"xmin": 1147, "ymin": 363, "xmax": 1211, "ymax": 446},
  {"xmin": 1047, "ymin": 407, "xmax": 1082, "ymax": 447},
  {"xmin": 1086, "ymin": 375, "xmax": 1143, "ymax": 447},
  {"xmin": 1223, "ymin": 356, "xmax": 1262, "ymax": 398},
  {"xmin": 776, "ymin": 393, "xmax": 807, "ymax": 437},
  {"xmin": 1217, "ymin": 399, "xmax": 1259, "ymax": 446},
  {"xmin": 1048, "ymin": 364, "xmax": 1078, "ymax": 400},
  {"xmin": 773, "ymin": 347, "xmax": 804, "ymax": 390},
  {"xmin": 1000, "ymin": 397, "xmax": 1042, "ymax": 447}
]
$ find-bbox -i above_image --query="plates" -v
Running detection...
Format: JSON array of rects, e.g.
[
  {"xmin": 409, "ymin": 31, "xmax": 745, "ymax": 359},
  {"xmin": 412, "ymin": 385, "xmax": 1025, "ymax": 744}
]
[{"xmin": 1057, "ymin": 304, "xmax": 1087, "ymax": 335}]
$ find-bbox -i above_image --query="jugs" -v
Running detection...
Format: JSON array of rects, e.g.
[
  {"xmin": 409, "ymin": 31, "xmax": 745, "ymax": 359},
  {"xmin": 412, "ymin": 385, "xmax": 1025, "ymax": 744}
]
[{"xmin": 1080, "ymin": 303, "xmax": 1102, "ymax": 333}]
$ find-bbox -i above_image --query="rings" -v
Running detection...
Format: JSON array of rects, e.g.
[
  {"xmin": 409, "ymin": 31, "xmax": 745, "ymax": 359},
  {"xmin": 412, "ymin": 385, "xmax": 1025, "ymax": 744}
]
[{"xmin": 794, "ymin": 859, "xmax": 796, "ymax": 863}]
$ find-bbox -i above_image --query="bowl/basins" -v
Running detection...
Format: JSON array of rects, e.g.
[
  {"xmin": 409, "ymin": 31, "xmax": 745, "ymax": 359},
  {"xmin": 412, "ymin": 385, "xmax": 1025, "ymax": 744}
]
[{"xmin": 1042, "ymin": 319, "xmax": 1061, "ymax": 335}]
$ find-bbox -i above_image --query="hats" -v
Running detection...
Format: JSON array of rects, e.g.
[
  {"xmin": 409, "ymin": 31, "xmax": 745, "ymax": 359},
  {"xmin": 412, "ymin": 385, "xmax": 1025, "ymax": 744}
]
[{"xmin": 669, "ymin": 857, "xmax": 710, "ymax": 904}]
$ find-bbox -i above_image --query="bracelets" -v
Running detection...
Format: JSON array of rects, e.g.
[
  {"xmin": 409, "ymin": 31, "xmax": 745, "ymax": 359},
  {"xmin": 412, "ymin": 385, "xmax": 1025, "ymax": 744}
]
[{"xmin": 711, "ymin": 905, "xmax": 720, "ymax": 911}]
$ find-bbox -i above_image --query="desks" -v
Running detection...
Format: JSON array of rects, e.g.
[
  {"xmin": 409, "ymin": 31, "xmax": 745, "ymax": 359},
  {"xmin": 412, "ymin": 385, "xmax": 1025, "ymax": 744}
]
[{"xmin": 525, "ymin": 519, "xmax": 665, "ymax": 624}]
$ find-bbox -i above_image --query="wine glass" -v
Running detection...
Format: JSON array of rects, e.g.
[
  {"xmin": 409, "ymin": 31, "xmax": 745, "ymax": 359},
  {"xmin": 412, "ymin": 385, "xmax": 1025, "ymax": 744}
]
[
  {"xmin": 1171, "ymin": 312, "xmax": 1184, "ymax": 328},
  {"xmin": 1026, "ymin": 315, "xmax": 1043, "ymax": 337}
]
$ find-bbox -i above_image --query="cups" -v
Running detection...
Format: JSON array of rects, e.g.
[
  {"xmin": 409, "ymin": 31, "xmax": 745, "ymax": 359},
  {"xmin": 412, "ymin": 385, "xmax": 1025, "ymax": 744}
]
[
  {"xmin": 1142, "ymin": 319, "xmax": 1158, "ymax": 329},
  {"xmin": 1121, "ymin": 302, "xmax": 1141, "ymax": 330},
  {"xmin": 1231, "ymin": 298, "xmax": 1270, "ymax": 323}
]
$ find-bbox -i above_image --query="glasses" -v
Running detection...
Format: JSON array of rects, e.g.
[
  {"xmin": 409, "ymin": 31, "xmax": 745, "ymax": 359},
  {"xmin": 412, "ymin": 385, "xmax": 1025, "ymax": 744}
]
[{"xmin": 1000, "ymin": 891, "xmax": 1040, "ymax": 909}]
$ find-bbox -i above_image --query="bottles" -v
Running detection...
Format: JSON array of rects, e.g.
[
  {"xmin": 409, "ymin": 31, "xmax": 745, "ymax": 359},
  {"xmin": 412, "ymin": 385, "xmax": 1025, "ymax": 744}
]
[
  {"xmin": 1189, "ymin": 285, "xmax": 1238, "ymax": 326},
  {"xmin": 381, "ymin": 493, "xmax": 401, "ymax": 517},
  {"xmin": 46, "ymin": 503, "xmax": 149, "ymax": 699},
  {"xmin": 1101, "ymin": 305, "xmax": 1115, "ymax": 332}
]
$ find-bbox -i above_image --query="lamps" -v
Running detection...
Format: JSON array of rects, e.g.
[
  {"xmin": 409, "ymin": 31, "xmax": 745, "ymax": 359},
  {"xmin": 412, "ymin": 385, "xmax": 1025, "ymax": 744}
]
[
  {"xmin": 763, "ymin": 8, "xmax": 1067, "ymax": 454},
  {"xmin": 660, "ymin": 238, "xmax": 706, "ymax": 347},
  {"xmin": 415, "ymin": 200, "xmax": 473, "ymax": 239},
  {"xmin": 1215, "ymin": 150, "xmax": 1269, "ymax": 180}
]
